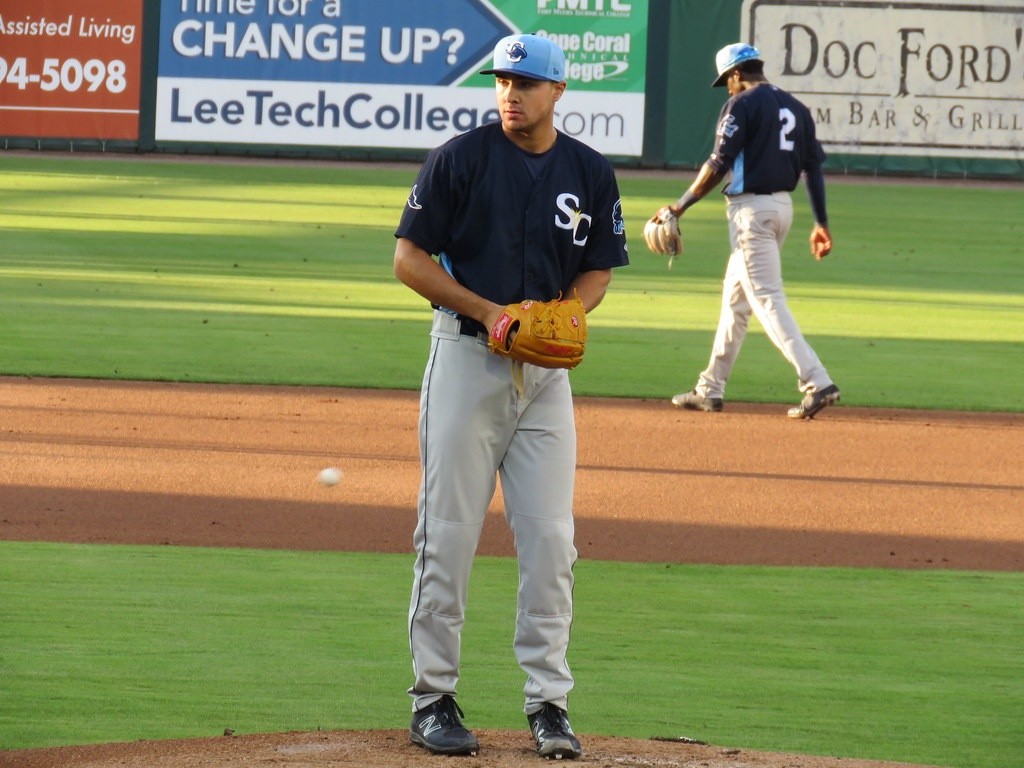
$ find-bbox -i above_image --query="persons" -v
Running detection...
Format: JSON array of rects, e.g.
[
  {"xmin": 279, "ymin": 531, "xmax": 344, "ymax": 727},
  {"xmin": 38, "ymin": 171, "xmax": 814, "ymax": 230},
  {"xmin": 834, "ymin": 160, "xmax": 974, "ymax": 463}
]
[
  {"xmin": 392, "ymin": 36, "xmax": 629, "ymax": 757},
  {"xmin": 643, "ymin": 42, "xmax": 842, "ymax": 418}
]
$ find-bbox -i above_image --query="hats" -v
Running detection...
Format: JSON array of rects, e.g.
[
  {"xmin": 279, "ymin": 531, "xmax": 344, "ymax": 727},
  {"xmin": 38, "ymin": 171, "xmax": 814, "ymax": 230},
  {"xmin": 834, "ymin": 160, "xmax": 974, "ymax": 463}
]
[
  {"xmin": 711, "ymin": 42, "xmax": 763, "ymax": 87},
  {"xmin": 479, "ymin": 33, "xmax": 564, "ymax": 82}
]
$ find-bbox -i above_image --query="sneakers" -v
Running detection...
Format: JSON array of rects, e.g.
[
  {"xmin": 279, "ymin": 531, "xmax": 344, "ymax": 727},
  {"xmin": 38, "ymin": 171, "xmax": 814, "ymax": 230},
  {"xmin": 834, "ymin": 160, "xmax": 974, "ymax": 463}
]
[
  {"xmin": 672, "ymin": 389, "xmax": 724, "ymax": 412},
  {"xmin": 526, "ymin": 702, "xmax": 582, "ymax": 759},
  {"xmin": 409, "ymin": 694, "xmax": 479, "ymax": 756},
  {"xmin": 788, "ymin": 384, "xmax": 842, "ymax": 418}
]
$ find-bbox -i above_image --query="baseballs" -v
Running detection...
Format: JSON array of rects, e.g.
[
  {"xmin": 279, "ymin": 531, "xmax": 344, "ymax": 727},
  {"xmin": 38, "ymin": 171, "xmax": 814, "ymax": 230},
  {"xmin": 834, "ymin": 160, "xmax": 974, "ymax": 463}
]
[{"xmin": 317, "ymin": 467, "xmax": 342, "ymax": 487}]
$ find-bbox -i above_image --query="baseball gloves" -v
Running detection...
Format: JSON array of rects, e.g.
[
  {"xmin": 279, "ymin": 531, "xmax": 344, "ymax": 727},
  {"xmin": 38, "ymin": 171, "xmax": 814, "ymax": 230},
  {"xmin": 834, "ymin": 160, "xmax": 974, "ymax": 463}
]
[
  {"xmin": 642, "ymin": 206, "xmax": 682, "ymax": 257},
  {"xmin": 486, "ymin": 298, "xmax": 587, "ymax": 371}
]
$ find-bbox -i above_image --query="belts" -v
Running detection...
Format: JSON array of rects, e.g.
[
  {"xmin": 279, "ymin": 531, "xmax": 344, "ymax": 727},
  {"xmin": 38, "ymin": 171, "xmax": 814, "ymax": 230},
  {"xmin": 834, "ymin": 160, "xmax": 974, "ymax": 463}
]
[
  {"xmin": 755, "ymin": 191, "xmax": 773, "ymax": 196},
  {"xmin": 459, "ymin": 322, "xmax": 479, "ymax": 338}
]
